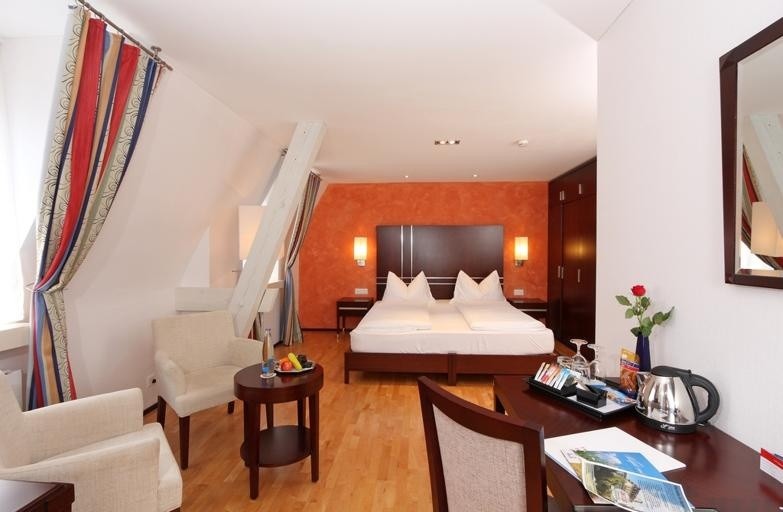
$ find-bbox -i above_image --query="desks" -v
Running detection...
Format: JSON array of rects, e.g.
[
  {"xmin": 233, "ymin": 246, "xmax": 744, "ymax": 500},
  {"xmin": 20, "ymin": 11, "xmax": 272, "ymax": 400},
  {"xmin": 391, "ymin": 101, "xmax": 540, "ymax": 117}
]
[{"xmin": 493, "ymin": 375, "xmax": 782, "ymax": 512}]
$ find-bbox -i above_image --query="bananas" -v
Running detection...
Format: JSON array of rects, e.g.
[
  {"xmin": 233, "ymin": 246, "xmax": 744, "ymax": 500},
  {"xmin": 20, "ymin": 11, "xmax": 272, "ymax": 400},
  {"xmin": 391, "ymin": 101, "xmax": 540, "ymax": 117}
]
[{"xmin": 287, "ymin": 352, "xmax": 302, "ymax": 370}]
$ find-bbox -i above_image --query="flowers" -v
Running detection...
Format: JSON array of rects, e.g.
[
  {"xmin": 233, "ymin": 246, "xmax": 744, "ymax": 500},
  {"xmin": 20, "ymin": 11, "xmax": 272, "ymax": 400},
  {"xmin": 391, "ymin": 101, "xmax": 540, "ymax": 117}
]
[{"xmin": 615, "ymin": 284, "xmax": 675, "ymax": 338}]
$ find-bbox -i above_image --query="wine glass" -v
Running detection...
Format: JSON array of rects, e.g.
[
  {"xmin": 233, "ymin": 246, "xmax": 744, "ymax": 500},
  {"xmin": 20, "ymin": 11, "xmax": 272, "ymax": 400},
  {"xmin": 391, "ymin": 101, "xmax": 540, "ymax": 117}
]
[
  {"xmin": 585, "ymin": 344, "xmax": 607, "ymax": 385},
  {"xmin": 570, "ymin": 339, "xmax": 588, "ymax": 364}
]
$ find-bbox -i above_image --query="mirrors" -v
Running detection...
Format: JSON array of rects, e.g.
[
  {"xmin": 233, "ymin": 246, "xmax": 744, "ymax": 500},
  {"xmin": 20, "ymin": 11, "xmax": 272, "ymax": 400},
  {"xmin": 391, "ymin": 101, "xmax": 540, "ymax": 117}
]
[{"xmin": 719, "ymin": 18, "xmax": 783, "ymax": 289}]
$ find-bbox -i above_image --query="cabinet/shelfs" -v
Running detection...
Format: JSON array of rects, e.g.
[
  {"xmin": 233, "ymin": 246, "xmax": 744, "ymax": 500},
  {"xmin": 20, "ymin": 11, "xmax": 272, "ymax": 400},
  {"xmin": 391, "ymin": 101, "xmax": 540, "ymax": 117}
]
[{"xmin": 546, "ymin": 156, "xmax": 597, "ymax": 363}]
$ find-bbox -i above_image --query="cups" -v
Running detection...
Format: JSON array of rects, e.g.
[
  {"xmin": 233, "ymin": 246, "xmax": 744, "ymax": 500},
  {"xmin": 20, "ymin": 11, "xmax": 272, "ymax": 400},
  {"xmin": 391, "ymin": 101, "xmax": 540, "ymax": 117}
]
[{"xmin": 557, "ymin": 356, "xmax": 590, "ymax": 385}]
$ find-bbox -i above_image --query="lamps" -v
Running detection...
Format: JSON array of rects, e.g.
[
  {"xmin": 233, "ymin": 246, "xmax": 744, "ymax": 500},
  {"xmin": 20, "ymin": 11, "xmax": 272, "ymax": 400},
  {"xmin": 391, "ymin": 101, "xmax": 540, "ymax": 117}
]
[
  {"xmin": 514, "ymin": 236, "xmax": 528, "ymax": 266},
  {"xmin": 353, "ymin": 237, "xmax": 368, "ymax": 266}
]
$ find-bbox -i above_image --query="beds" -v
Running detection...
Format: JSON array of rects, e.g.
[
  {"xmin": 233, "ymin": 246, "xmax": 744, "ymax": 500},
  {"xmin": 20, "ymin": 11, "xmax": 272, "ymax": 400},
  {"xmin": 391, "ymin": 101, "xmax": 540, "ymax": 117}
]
[{"xmin": 344, "ymin": 223, "xmax": 559, "ymax": 384}]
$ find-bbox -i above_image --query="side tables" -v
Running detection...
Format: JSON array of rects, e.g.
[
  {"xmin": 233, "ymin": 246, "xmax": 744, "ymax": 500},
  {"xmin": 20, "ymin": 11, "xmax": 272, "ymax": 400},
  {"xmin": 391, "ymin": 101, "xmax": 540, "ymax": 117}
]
[{"xmin": 234, "ymin": 361, "xmax": 324, "ymax": 500}]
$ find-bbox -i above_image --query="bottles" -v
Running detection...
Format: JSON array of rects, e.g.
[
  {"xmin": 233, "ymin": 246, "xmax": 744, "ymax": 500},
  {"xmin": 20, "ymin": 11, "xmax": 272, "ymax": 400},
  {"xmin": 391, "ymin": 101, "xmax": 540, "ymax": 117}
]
[{"xmin": 262, "ymin": 328, "xmax": 275, "ymax": 377}]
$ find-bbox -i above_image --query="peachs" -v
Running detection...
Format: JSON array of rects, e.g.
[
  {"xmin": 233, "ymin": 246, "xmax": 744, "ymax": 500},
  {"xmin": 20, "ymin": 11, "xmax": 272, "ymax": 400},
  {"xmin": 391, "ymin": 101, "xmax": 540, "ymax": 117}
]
[{"xmin": 282, "ymin": 361, "xmax": 292, "ymax": 371}]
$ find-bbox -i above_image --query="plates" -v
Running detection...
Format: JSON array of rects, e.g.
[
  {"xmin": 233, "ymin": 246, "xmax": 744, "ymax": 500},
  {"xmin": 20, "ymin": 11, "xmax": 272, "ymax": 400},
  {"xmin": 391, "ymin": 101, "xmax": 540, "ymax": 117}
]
[{"xmin": 274, "ymin": 359, "xmax": 315, "ymax": 373}]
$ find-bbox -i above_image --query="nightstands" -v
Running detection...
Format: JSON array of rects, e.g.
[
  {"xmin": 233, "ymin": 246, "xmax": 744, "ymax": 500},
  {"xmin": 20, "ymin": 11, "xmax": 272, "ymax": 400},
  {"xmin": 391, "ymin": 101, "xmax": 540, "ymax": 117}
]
[
  {"xmin": 507, "ymin": 297, "xmax": 548, "ymax": 320},
  {"xmin": 336, "ymin": 297, "xmax": 374, "ymax": 339}
]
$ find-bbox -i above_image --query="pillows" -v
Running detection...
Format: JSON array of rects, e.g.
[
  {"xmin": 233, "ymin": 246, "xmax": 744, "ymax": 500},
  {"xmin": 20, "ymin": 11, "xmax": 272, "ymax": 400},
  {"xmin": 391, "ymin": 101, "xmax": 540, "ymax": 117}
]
[
  {"xmin": 381, "ymin": 270, "xmax": 436, "ymax": 303},
  {"xmin": 451, "ymin": 270, "xmax": 504, "ymax": 304}
]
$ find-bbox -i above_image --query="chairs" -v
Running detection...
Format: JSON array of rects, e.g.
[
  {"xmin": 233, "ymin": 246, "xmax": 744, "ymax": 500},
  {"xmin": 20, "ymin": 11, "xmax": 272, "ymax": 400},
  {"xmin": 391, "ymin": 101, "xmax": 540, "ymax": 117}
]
[
  {"xmin": 1, "ymin": 370, "xmax": 183, "ymax": 512},
  {"xmin": 417, "ymin": 376, "xmax": 548, "ymax": 512},
  {"xmin": 151, "ymin": 310, "xmax": 274, "ymax": 470}
]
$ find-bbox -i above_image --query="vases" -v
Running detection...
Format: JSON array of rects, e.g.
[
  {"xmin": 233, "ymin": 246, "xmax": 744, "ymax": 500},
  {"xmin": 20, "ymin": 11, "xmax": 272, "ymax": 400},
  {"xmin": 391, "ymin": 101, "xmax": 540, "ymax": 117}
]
[{"xmin": 636, "ymin": 333, "xmax": 650, "ymax": 372}]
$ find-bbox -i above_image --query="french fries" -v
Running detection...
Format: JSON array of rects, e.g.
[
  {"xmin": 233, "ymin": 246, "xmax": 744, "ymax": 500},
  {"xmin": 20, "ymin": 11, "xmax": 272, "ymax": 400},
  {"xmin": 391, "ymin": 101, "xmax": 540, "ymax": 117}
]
[{"xmin": 620, "ymin": 370, "xmax": 637, "ymax": 392}]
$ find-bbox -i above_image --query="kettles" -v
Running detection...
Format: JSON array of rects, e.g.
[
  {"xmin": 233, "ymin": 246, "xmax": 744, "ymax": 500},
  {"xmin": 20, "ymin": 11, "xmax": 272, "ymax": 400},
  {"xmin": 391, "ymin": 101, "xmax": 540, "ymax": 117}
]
[{"xmin": 634, "ymin": 366, "xmax": 720, "ymax": 434}]
